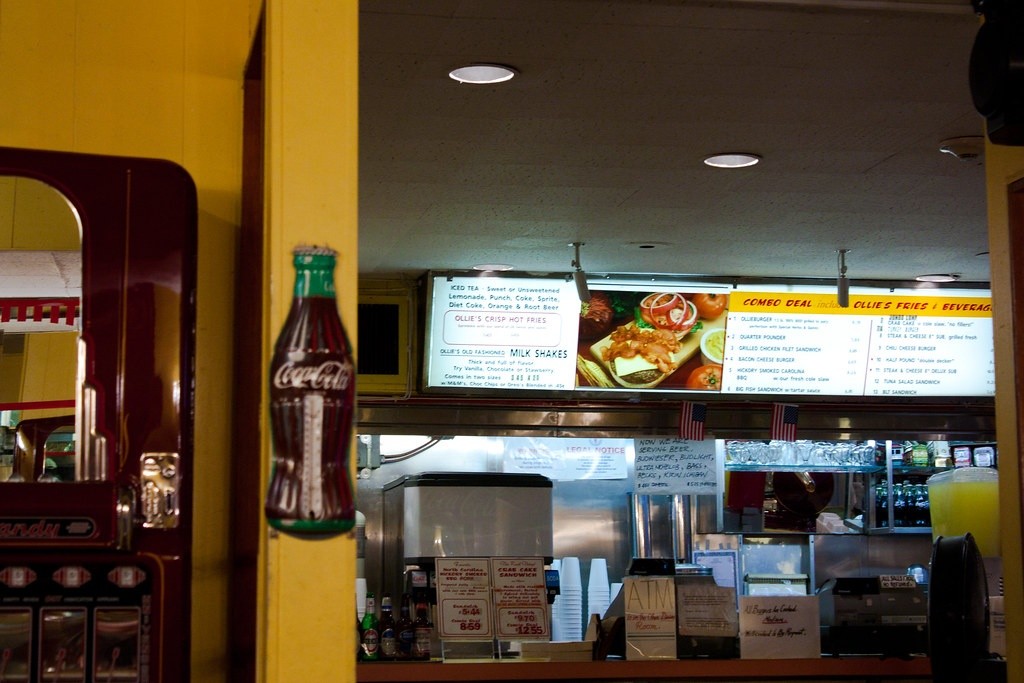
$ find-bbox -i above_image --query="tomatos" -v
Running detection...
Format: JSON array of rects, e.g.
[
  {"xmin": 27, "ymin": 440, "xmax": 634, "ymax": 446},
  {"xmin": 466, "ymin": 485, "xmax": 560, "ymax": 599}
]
[
  {"xmin": 685, "ymin": 363, "xmax": 722, "ymax": 391},
  {"xmin": 692, "ymin": 293, "xmax": 728, "ymax": 320}
]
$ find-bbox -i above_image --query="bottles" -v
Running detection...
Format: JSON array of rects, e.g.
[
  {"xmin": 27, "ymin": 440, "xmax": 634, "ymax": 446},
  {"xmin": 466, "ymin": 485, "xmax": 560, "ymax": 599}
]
[
  {"xmin": 265, "ymin": 247, "xmax": 355, "ymax": 535},
  {"xmin": 876, "ymin": 480, "xmax": 932, "ymax": 527},
  {"xmin": 414, "ymin": 592, "xmax": 431, "ymax": 661},
  {"xmin": 360, "ymin": 591, "xmax": 379, "ymax": 660},
  {"xmin": 397, "ymin": 593, "xmax": 413, "ymax": 661},
  {"xmin": 378, "ymin": 593, "xmax": 396, "ymax": 661}
]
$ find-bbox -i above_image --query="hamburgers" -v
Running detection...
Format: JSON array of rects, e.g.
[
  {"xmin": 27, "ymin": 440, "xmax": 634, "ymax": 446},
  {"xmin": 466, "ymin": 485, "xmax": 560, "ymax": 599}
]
[{"xmin": 602, "ymin": 289, "xmax": 704, "ymax": 389}]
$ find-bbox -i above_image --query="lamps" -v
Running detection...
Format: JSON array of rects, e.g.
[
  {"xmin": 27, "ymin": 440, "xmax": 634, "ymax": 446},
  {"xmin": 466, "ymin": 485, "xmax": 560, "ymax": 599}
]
[
  {"xmin": 837, "ymin": 248, "xmax": 849, "ymax": 308},
  {"xmin": 567, "ymin": 243, "xmax": 592, "ymax": 302}
]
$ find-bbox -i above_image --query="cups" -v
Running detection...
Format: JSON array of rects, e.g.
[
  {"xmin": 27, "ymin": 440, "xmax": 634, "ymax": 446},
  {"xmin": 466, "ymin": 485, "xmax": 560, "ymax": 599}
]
[
  {"xmin": 729, "ymin": 441, "xmax": 873, "ymax": 466},
  {"xmin": 551, "ymin": 556, "xmax": 623, "ymax": 642}
]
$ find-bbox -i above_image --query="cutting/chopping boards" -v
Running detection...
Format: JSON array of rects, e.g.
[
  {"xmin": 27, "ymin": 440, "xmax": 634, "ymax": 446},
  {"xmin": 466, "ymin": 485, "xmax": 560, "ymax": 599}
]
[{"xmin": 590, "ymin": 319, "xmax": 701, "ymax": 389}]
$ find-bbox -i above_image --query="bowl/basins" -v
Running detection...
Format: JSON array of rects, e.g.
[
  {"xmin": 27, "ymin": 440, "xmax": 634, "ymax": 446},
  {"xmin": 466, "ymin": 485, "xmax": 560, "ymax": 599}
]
[{"xmin": 700, "ymin": 328, "xmax": 725, "ymax": 366}]
